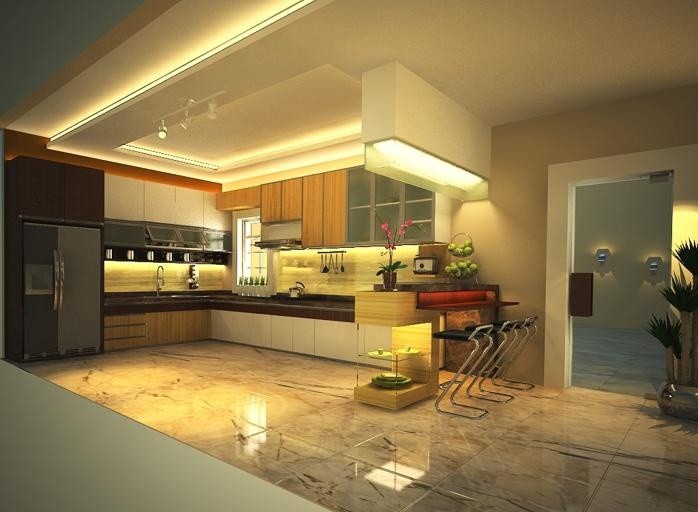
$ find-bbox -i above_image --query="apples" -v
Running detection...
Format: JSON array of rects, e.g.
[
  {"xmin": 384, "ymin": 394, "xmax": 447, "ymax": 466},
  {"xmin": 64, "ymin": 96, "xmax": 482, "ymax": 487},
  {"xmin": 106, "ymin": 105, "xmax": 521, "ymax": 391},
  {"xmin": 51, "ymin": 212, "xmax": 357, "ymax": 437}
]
[{"xmin": 444, "ymin": 241, "xmax": 478, "ymax": 278}]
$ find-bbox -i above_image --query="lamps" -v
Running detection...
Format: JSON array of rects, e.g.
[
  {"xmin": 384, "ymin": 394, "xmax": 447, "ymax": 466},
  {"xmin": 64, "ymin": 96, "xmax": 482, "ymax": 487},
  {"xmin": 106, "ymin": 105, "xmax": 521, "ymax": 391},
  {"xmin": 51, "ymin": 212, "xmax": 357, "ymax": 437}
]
[{"xmin": 156, "ymin": 99, "xmax": 218, "ymax": 140}]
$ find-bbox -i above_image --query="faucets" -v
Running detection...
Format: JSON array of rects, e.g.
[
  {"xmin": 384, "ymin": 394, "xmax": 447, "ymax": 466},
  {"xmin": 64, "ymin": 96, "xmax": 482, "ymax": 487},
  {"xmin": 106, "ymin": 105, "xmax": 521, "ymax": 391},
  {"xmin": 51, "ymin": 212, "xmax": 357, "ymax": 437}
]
[{"xmin": 156, "ymin": 266, "xmax": 163, "ymax": 295}]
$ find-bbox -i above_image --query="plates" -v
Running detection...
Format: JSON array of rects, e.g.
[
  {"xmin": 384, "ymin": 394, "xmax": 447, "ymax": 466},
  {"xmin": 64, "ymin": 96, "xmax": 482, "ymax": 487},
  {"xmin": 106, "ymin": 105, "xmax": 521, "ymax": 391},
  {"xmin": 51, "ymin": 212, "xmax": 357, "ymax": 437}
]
[{"xmin": 368, "ymin": 349, "xmax": 419, "ymax": 360}]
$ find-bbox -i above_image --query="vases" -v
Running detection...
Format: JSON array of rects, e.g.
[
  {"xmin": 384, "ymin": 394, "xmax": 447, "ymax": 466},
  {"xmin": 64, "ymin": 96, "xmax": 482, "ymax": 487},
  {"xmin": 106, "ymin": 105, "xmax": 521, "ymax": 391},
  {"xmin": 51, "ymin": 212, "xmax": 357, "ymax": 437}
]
[{"xmin": 382, "ymin": 272, "xmax": 397, "ymax": 291}]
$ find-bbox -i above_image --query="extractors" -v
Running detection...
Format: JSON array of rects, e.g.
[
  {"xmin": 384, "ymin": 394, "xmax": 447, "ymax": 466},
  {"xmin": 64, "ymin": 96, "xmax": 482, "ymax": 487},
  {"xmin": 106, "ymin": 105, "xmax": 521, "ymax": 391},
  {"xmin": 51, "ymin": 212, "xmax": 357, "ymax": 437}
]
[{"xmin": 255, "ymin": 240, "xmax": 304, "ymax": 251}]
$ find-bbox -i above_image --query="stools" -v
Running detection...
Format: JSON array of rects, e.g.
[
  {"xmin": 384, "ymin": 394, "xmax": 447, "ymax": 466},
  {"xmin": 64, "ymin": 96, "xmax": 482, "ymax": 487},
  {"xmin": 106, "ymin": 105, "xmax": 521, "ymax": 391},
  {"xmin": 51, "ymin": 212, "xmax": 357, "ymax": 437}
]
[{"xmin": 432, "ymin": 314, "xmax": 538, "ymax": 420}]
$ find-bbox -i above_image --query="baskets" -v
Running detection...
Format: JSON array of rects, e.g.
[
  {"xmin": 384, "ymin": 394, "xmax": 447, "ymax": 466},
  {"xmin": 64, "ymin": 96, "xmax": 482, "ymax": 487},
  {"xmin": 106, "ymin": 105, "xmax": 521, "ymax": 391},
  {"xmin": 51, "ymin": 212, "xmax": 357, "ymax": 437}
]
[
  {"xmin": 444, "ymin": 269, "xmax": 479, "ymax": 280},
  {"xmin": 448, "ymin": 250, "xmax": 473, "ymax": 257}
]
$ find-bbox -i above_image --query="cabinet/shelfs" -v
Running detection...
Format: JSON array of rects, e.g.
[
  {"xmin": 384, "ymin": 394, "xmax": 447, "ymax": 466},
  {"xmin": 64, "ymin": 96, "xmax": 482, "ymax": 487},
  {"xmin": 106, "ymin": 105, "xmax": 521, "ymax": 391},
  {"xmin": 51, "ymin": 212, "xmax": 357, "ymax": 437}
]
[
  {"xmin": 216, "ymin": 186, "xmax": 261, "ymax": 212},
  {"xmin": 102, "ymin": 309, "xmax": 211, "ymax": 352},
  {"xmin": 314, "ymin": 319, "xmax": 393, "ymax": 369},
  {"xmin": 210, "ymin": 308, "xmax": 271, "ymax": 348},
  {"xmin": 301, "ymin": 169, "xmax": 349, "ymax": 248},
  {"xmin": 271, "ymin": 315, "xmax": 314, "ymax": 357},
  {"xmin": 260, "ymin": 177, "xmax": 302, "ymax": 224}
]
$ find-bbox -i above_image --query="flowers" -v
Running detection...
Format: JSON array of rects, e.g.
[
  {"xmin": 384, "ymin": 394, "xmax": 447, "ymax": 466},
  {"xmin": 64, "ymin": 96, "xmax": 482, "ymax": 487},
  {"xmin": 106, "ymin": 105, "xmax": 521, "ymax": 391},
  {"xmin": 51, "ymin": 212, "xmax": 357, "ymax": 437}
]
[{"xmin": 374, "ymin": 209, "xmax": 422, "ymax": 276}]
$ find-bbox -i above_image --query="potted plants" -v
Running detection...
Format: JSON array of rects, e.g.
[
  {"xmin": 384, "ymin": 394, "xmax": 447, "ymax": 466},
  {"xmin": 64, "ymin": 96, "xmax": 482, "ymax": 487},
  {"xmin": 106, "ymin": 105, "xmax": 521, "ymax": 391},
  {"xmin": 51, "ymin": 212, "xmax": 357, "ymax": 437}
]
[
  {"xmin": 237, "ymin": 275, "xmax": 272, "ymax": 298},
  {"xmin": 643, "ymin": 236, "xmax": 698, "ymax": 421}
]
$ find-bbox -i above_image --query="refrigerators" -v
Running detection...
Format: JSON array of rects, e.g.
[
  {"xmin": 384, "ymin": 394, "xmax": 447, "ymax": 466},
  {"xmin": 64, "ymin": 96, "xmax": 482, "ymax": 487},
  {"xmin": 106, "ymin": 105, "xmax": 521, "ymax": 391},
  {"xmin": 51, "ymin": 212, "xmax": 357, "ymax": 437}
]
[{"xmin": 21, "ymin": 221, "xmax": 102, "ymax": 362}]
